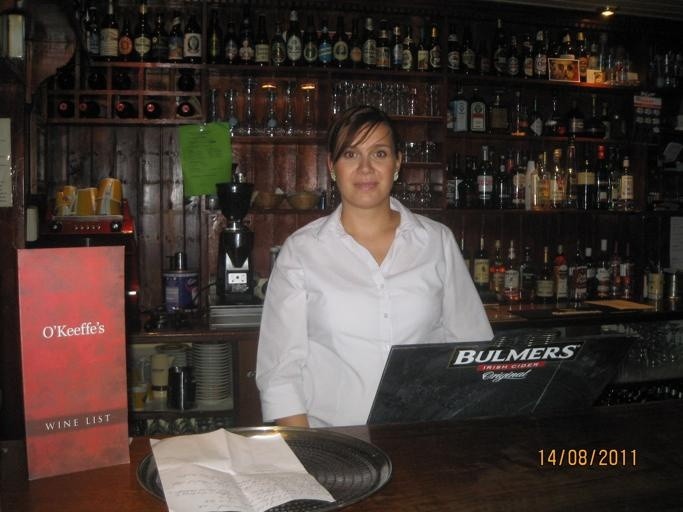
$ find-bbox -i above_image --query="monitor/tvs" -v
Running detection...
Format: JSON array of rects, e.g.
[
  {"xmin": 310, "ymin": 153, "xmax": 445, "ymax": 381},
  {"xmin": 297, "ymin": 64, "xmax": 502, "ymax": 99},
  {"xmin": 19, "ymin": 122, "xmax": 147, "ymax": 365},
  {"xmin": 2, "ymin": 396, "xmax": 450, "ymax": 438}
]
[{"xmin": 367, "ymin": 334, "xmax": 635, "ymax": 426}]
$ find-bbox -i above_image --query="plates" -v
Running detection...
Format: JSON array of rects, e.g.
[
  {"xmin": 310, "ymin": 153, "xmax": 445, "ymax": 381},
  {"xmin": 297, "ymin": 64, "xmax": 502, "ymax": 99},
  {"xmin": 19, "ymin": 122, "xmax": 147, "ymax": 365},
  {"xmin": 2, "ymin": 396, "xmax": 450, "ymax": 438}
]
[
  {"xmin": 189, "ymin": 339, "xmax": 230, "ymax": 405},
  {"xmin": 153, "ymin": 344, "xmax": 188, "ymax": 369}
]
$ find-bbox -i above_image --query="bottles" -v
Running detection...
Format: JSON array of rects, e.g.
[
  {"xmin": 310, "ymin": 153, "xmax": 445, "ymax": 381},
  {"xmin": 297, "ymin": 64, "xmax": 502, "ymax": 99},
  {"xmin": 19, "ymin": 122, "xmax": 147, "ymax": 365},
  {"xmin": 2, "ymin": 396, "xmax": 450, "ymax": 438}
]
[
  {"xmin": 269, "ymin": 244, "xmax": 281, "ymax": 276},
  {"xmin": 55, "ymin": 73, "xmax": 326, "ymax": 138},
  {"xmin": 459, "ymin": 230, "xmax": 679, "ymax": 305},
  {"xmin": 200, "ymin": 193, "xmax": 216, "ymax": 211},
  {"xmin": 320, "ymin": 182, "xmax": 338, "ymax": 211},
  {"xmin": 72, "ymin": 0, "xmax": 683, "ymax": 92},
  {"xmin": 445, "ymin": 84, "xmax": 636, "ymax": 213}
]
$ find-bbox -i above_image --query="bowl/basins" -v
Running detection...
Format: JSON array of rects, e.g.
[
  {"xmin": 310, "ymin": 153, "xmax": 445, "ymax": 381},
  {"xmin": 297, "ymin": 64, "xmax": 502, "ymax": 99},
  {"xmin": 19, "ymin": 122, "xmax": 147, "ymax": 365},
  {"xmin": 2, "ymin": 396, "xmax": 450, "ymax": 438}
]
[
  {"xmin": 256, "ymin": 193, "xmax": 285, "ymax": 211},
  {"xmin": 288, "ymin": 194, "xmax": 319, "ymax": 210}
]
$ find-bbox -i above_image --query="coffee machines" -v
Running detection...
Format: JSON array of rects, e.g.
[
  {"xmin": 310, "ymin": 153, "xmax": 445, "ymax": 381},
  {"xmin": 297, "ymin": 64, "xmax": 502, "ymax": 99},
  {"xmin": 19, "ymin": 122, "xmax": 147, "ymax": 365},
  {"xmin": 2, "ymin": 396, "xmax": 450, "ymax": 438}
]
[{"xmin": 214, "ymin": 181, "xmax": 253, "ymax": 300}]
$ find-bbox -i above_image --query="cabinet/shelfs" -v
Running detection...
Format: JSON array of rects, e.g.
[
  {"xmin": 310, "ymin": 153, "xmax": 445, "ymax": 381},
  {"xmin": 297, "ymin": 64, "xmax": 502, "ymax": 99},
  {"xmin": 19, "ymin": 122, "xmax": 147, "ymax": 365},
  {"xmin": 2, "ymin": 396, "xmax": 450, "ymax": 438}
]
[
  {"xmin": 30, "ymin": 1, "xmax": 207, "ymax": 127},
  {"xmin": 482, "ymin": 297, "xmax": 682, "ymax": 408},
  {"xmin": 124, "ymin": 329, "xmax": 266, "ymax": 435},
  {"xmin": 651, "ymin": 21, "xmax": 683, "ymax": 218},
  {"xmin": 207, "ymin": 0, "xmax": 448, "ymax": 216},
  {"xmin": 448, "ymin": 9, "xmax": 650, "ymax": 215}
]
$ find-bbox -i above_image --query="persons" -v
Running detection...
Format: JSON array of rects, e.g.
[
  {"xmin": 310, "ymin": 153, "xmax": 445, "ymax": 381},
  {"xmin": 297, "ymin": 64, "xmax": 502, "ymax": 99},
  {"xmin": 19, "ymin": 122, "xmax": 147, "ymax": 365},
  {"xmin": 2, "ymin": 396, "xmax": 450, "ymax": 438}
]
[{"xmin": 255, "ymin": 105, "xmax": 495, "ymax": 430}]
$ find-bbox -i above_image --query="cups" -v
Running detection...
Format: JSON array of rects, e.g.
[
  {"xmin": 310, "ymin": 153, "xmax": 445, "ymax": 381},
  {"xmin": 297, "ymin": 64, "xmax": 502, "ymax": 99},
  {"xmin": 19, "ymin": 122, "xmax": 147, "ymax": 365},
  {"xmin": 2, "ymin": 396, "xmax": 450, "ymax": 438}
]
[
  {"xmin": 404, "ymin": 140, "xmax": 438, "ymax": 163},
  {"xmin": 149, "ymin": 353, "xmax": 171, "ymax": 399},
  {"xmin": 629, "ymin": 324, "xmax": 678, "ymax": 368},
  {"xmin": 389, "ymin": 182, "xmax": 434, "ymax": 209},
  {"xmin": 166, "ymin": 367, "xmax": 196, "ymax": 409},
  {"xmin": 126, "ymin": 387, "xmax": 146, "ymax": 409},
  {"xmin": 52, "ymin": 176, "xmax": 123, "ymax": 217},
  {"xmin": 330, "ymin": 81, "xmax": 442, "ymax": 116}
]
